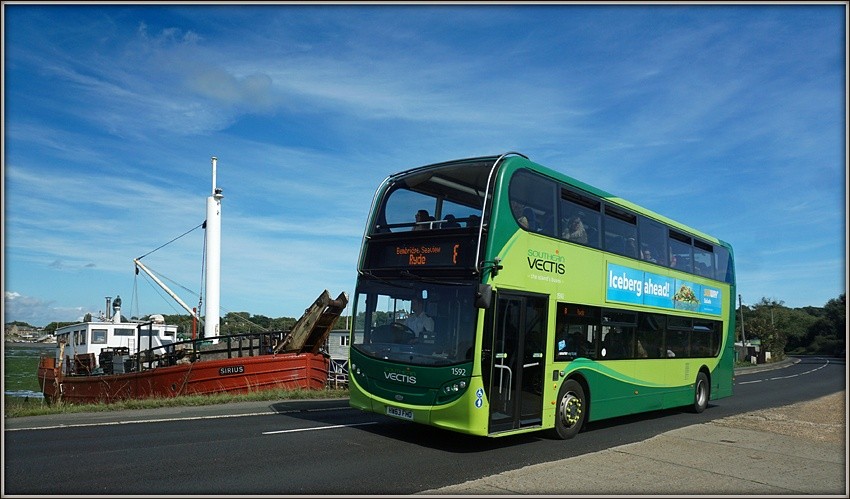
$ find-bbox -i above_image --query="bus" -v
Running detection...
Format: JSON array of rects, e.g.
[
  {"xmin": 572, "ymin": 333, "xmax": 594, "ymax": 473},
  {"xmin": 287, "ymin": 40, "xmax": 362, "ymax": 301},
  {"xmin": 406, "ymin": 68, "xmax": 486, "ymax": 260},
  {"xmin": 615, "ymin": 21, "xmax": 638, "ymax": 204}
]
[{"xmin": 345, "ymin": 152, "xmax": 739, "ymax": 442}]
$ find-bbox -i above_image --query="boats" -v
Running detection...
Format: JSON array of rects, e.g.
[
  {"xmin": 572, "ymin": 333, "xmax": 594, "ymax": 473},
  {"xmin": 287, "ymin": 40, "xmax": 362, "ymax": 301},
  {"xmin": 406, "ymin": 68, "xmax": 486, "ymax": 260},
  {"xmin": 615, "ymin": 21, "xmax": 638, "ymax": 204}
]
[{"xmin": 35, "ymin": 155, "xmax": 349, "ymax": 407}]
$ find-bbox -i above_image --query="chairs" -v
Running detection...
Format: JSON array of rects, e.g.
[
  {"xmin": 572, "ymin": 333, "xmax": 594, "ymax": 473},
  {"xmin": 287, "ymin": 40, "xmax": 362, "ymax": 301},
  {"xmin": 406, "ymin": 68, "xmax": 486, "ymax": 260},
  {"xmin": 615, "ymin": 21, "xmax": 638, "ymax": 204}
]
[{"xmin": 409, "ymin": 204, "xmax": 733, "ymax": 281}]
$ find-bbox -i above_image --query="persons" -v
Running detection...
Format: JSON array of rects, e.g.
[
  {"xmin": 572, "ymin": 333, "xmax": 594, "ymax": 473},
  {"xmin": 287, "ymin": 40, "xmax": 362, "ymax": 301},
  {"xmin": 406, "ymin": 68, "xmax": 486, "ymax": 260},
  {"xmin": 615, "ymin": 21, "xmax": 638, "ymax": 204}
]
[
  {"xmin": 399, "ymin": 296, "xmax": 434, "ymax": 344},
  {"xmin": 511, "ymin": 200, "xmax": 704, "ymax": 278},
  {"xmin": 411, "ymin": 210, "xmax": 481, "ymax": 231},
  {"xmin": 555, "ymin": 315, "xmax": 715, "ymax": 359}
]
